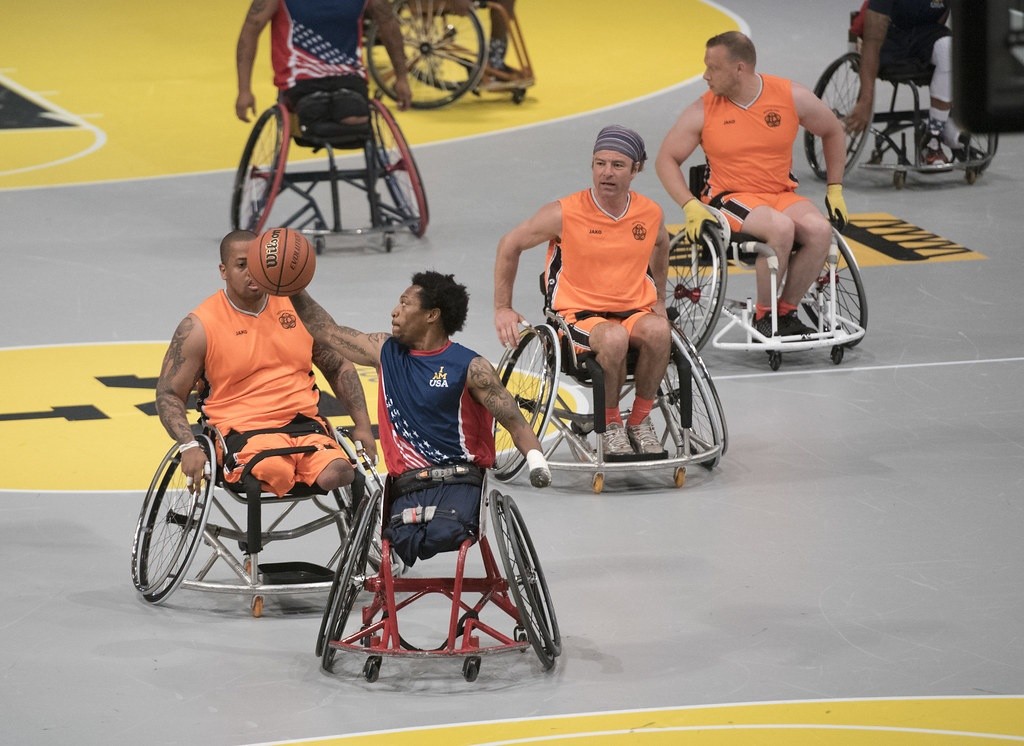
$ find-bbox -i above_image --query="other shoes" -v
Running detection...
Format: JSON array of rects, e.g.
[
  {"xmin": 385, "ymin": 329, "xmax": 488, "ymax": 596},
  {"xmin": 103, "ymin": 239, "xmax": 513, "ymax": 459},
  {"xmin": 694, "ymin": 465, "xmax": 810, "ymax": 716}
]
[{"xmin": 919, "ymin": 128, "xmax": 947, "ymax": 166}]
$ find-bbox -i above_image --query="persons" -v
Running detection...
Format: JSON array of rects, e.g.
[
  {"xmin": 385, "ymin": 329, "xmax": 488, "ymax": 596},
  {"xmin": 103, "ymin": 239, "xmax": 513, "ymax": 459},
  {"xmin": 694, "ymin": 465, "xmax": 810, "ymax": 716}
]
[
  {"xmin": 236, "ymin": 0, "xmax": 411, "ymax": 128},
  {"xmin": 155, "ymin": 228, "xmax": 378, "ymax": 497},
  {"xmin": 492, "ymin": 125, "xmax": 671, "ymax": 457},
  {"xmin": 410, "ymin": 0, "xmax": 524, "ymax": 84},
  {"xmin": 288, "ymin": 271, "xmax": 551, "ymax": 562},
  {"xmin": 842, "ymin": 0, "xmax": 983, "ymax": 167},
  {"xmin": 655, "ymin": 32, "xmax": 847, "ymax": 345}
]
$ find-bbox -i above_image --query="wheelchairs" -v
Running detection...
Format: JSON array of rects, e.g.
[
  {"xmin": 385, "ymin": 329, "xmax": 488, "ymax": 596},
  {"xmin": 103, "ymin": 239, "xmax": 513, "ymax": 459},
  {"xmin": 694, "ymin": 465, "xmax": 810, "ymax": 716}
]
[
  {"xmin": 366, "ymin": 1, "xmax": 534, "ymax": 114},
  {"xmin": 481, "ymin": 318, "xmax": 729, "ymax": 489},
  {"xmin": 318, "ymin": 494, "xmax": 563, "ymax": 679},
  {"xmin": 663, "ymin": 219, "xmax": 869, "ymax": 374},
  {"xmin": 800, "ymin": 55, "xmax": 998, "ymax": 189},
  {"xmin": 231, "ymin": 101, "xmax": 432, "ymax": 258},
  {"xmin": 127, "ymin": 425, "xmax": 394, "ymax": 605}
]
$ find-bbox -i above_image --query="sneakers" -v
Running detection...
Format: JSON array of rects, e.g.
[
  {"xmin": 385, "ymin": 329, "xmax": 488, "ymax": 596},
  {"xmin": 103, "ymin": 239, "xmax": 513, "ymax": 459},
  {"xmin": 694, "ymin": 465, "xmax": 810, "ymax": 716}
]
[
  {"xmin": 603, "ymin": 423, "xmax": 635, "ymax": 456},
  {"xmin": 751, "ymin": 310, "xmax": 818, "ymax": 343},
  {"xmin": 626, "ymin": 415, "xmax": 665, "ymax": 454}
]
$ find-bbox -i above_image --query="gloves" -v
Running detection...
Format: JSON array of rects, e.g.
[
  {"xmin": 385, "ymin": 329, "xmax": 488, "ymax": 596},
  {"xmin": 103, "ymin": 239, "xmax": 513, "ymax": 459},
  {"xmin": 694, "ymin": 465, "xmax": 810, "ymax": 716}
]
[
  {"xmin": 825, "ymin": 182, "xmax": 849, "ymax": 233},
  {"xmin": 681, "ymin": 198, "xmax": 722, "ymax": 246}
]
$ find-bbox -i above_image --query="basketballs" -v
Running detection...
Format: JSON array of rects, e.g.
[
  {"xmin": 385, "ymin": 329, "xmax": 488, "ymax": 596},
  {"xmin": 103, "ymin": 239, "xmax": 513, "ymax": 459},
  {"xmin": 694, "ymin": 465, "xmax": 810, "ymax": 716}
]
[{"xmin": 247, "ymin": 228, "xmax": 316, "ymax": 296}]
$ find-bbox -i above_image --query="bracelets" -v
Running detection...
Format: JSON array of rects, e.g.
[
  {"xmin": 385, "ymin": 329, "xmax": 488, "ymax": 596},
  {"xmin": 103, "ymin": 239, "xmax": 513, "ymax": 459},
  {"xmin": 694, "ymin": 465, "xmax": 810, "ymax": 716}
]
[{"xmin": 179, "ymin": 441, "xmax": 199, "ymax": 453}]
